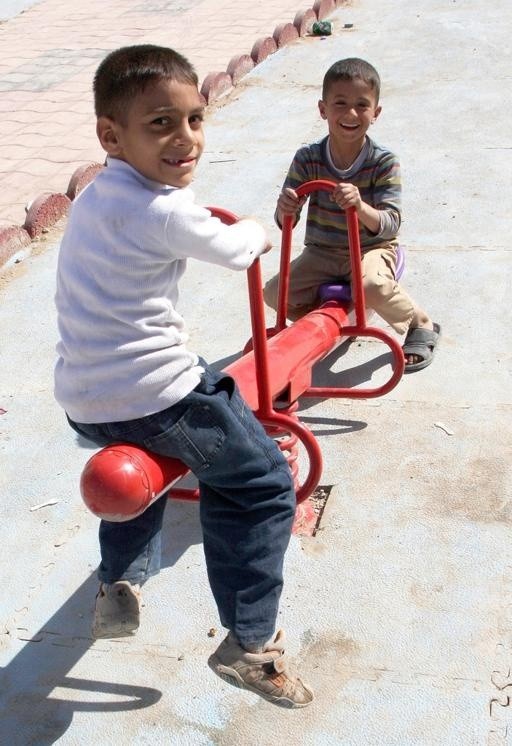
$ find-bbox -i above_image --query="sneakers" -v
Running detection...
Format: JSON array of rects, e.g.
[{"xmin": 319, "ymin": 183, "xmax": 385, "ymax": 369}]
[
  {"xmin": 206, "ymin": 630, "xmax": 315, "ymax": 712},
  {"xmin": 90, "ymin": 576, "xmax": 146, "ymax": 638}
]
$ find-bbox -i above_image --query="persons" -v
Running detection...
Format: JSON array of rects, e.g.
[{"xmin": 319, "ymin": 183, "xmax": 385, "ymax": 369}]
[
  {"xmin": 260, "ymin": 53, "xmax": 442, "ymax": 374},
  {"xmin": 51, "ymin": 41, "xmax": 317, "ymax": 713}
]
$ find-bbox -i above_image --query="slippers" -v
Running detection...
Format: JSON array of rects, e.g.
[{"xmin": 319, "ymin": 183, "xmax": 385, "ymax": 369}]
[{"xmin": 400, "ymin": 321, "xmax": 442, "ymax": 374}]
